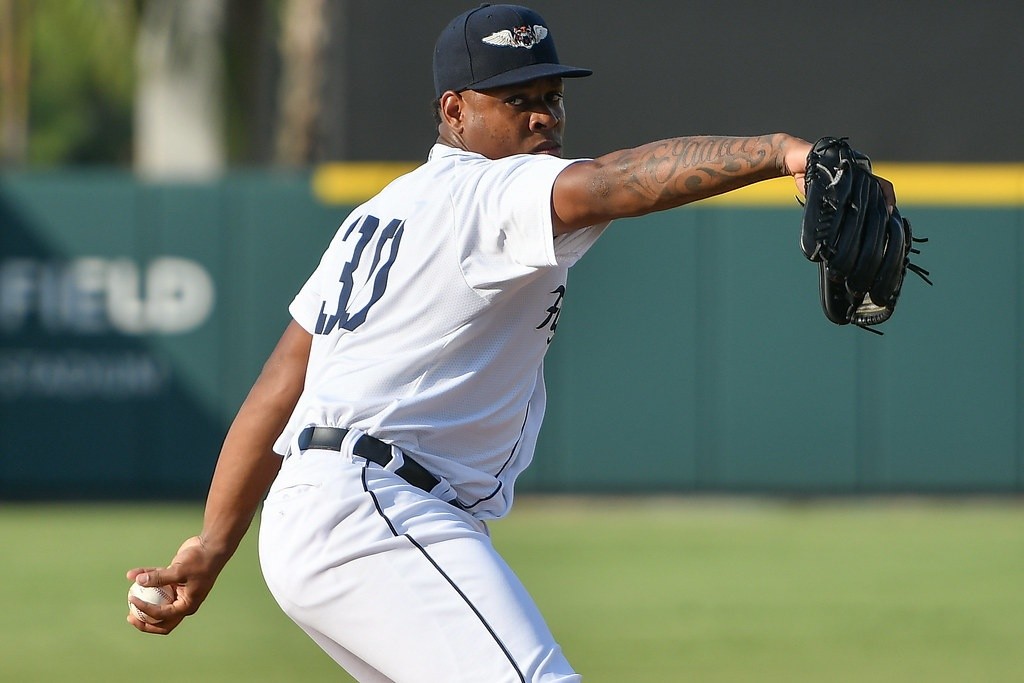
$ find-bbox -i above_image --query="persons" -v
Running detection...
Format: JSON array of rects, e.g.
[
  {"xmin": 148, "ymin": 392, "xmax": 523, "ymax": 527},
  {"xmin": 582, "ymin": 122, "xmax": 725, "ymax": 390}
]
[{"xmin": 128, "ymin": 2, "xmax": 913, "ymax": 683}]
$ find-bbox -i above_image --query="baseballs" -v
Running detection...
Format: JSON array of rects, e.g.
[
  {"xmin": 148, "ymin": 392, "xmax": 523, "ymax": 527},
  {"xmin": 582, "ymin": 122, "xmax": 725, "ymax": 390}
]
[{"xmin": 126, "ymin": 579, "xmax": 176, "ymax": 626}]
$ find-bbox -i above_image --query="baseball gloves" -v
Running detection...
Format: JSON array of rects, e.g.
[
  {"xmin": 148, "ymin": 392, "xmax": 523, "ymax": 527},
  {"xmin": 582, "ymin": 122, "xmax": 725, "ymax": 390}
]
[{"xmin": 795, "ymin": 135, "xmax": 915, "ymax": 328}]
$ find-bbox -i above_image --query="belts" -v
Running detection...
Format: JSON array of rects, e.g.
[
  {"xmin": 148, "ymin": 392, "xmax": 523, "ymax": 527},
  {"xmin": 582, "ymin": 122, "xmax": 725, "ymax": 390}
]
[{"xmin": 286, "ymin": 427, "xmax": 464, "ymax": 511}]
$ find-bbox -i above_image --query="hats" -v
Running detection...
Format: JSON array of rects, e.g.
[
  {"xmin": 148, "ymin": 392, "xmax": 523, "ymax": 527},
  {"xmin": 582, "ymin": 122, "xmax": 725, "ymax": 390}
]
[{"xmin": 433, "ymin": 3, "xmax": 593, "ymax": 99}]
